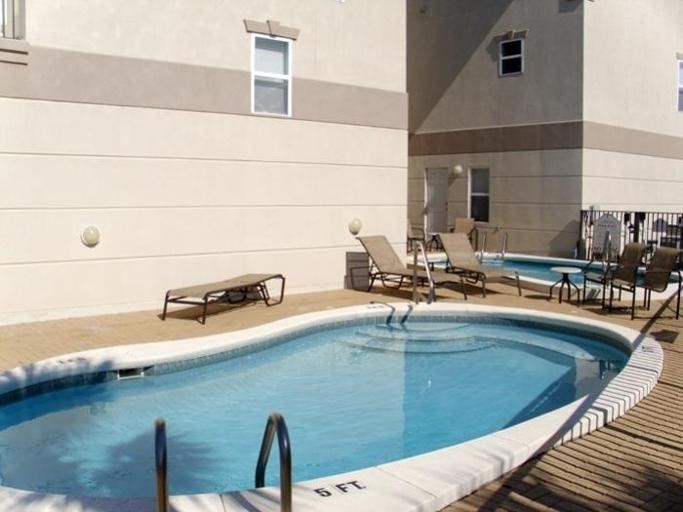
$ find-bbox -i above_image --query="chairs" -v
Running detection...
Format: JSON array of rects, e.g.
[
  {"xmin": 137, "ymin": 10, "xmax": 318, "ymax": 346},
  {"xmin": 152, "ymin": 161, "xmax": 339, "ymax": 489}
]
[
  {"xmin": 349, "ymin": 217, "xmax": 522, "ymax": 304},
  {"xmin": 160, "ymin": 272, "xmax": 286, "ymax": 325},
  {"xmin": 583, "ymin": 242, "xmax": 683, "ymax": 321}
]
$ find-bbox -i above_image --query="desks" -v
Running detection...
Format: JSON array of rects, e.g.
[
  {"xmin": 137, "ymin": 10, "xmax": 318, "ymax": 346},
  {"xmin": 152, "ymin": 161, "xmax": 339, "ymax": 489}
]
[{"xmin": 547, "ymin": 266, "xmax": 581, "ymax": 305}]
